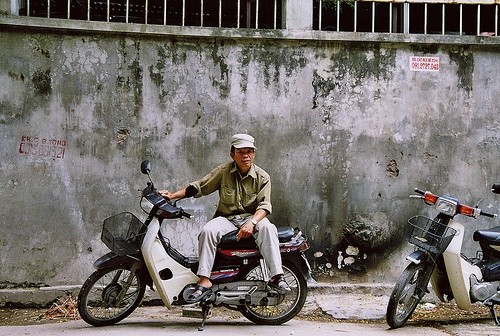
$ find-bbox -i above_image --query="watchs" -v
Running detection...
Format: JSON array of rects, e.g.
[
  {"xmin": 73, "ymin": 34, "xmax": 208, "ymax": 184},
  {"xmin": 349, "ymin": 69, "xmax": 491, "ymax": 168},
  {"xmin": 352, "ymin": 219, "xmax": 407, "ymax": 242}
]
[{"xmin": 250, "ymin": 219, "xmax": 257, "ymax": 227}]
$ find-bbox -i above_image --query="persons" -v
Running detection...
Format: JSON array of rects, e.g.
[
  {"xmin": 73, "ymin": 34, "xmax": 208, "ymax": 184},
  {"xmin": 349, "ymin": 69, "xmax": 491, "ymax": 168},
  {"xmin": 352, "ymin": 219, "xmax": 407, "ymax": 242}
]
[{"xmin": 158, "ymin": 133, "xmax": 291, "ymax": 303}]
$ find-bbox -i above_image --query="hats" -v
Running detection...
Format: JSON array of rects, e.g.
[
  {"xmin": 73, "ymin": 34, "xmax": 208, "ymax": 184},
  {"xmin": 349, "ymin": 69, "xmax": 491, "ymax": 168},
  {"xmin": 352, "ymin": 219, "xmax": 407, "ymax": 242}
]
[{"xmin": 231, "ymin": 134, "xmax": 257, "ymax": 149}]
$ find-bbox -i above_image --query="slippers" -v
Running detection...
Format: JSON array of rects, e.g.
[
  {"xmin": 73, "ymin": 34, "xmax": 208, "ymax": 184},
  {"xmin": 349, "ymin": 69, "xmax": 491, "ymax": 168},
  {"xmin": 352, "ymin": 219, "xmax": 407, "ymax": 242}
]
[
  {"xmin": 186, "ymin": 285, "xmax": 211, "ymax": 303},
  {"xmin": 268, "ymin": 276, "xmax": 292, "ymax": 291}
]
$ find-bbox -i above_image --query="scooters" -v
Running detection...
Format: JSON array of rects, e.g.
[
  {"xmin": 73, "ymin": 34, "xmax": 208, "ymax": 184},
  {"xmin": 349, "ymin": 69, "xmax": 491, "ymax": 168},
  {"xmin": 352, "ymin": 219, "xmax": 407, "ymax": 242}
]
[
  {"xmin": 386, "ymin": 182, "xmax": 500, "ymax": 329},
  {"xmin": 77, "ymin": 159, "xmax": 312, "ymax": 331}
]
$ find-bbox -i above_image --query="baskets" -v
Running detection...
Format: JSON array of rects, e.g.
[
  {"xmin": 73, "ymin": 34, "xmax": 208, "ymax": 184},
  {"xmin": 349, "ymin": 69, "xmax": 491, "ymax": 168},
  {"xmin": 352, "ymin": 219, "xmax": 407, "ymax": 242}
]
[
  {"xmin": 101, "ymin": 211, "xmax": 148, "ymax": 257},
  {"xmin": 405, "ymin": 215, "xmax": 457, "ymax": 254}
]
